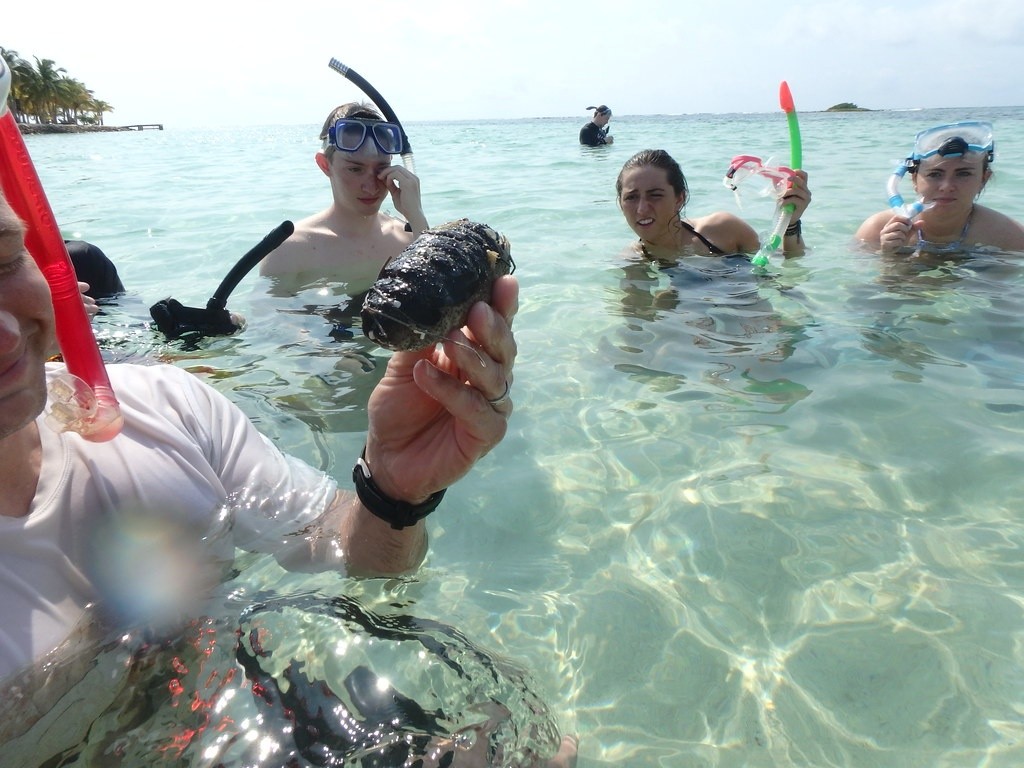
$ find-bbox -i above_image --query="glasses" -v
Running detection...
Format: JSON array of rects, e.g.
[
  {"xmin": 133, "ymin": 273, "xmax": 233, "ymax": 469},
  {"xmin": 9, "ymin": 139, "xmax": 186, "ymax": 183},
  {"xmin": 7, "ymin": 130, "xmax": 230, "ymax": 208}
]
[
  {"xmin": 322, "ymin": 116, "xmax": 403, "ymax": 154},
  {"xmin": 723, "ymin": 154, "xmax": 795, "ymax": 199},
  {"xmin": 913, "ymin": 121, "xmax": 995, "ymax": 164}
]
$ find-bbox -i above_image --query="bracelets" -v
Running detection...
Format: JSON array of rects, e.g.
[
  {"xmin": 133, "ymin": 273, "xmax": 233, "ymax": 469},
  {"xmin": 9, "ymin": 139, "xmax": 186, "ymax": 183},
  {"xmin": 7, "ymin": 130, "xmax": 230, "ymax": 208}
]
[
  {"xmin": 784, "ymin": 220, "xmax": 802, "ymax": 244},
  {"xmin": 354, "ymin": 445, "xmax": 446, "ymax": 530}
]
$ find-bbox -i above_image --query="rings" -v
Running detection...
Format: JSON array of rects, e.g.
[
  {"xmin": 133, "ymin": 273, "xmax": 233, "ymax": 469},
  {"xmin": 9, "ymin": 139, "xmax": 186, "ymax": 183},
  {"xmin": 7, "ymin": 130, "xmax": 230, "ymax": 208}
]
[{"xmin": 488, "ymin": 380, "xmax": 510, "ymax": 406}]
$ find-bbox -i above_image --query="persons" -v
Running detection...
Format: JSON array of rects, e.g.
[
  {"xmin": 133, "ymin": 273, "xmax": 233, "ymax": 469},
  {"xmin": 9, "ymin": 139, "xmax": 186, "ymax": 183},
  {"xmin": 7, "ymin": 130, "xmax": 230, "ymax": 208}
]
[
  {"xmin": 855, "ymin": 121, "xmax": 1024, "ymax": 254},
  {"xmin": 580, "ymin": 105, "xmax": 613, "ymax": 145},
  {"xmin": 0, "ymin": 187, "xmax": 517, "ymax": 691},
  {"xmin": 260, "ymin": 103, "xmax": 429, "ymax": 273},
  {"xmin": 64, "ymin": 240, "xmax": 126, "ymax": 323},
  {"xmin": 616, "ymin": 150, "xmax": 811, "ymax": 257}
]
